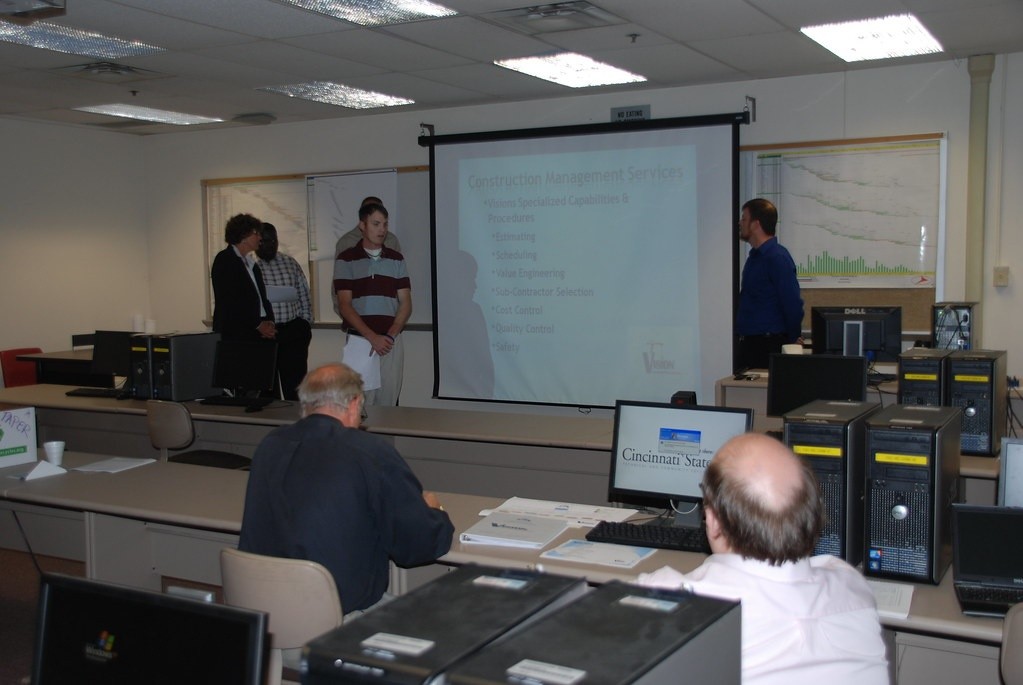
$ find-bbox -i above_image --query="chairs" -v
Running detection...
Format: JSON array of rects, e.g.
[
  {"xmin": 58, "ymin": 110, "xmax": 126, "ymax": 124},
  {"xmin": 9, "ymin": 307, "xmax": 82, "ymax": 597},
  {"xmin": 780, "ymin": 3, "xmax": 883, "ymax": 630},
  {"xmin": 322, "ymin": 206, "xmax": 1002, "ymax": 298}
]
[
  {"xmin": 219, "ymin": 547, "xmax": 344, "ymax": 685},
  {"xmin": 145, "ymin": 399, "xmax": 251, "ymax": 471}
]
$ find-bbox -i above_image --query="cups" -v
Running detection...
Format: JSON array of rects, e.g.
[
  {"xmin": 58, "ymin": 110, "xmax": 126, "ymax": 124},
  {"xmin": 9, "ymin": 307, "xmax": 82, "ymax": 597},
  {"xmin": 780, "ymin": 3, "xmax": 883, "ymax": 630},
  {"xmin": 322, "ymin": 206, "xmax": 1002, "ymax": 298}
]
[{"xmin": 45, "ymin": 441, "xmax": 65, "ymax": 464}]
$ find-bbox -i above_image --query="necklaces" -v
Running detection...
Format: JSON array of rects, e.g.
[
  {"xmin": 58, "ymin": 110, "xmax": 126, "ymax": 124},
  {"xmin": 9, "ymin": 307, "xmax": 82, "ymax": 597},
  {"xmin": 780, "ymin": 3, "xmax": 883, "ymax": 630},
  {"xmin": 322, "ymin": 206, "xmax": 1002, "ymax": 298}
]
[{"xmin": 366, "ymin": 248, "xmax": 383, "ymax": 257}]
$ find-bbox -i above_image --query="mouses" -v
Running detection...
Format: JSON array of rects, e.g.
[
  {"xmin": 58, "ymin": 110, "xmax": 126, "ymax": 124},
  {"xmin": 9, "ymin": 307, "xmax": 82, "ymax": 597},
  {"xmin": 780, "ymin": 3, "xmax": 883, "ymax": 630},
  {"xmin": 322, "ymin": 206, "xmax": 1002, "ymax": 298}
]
[{"xmin": 244, "ymin": 405, "xmax": 262, "ymax": 412}]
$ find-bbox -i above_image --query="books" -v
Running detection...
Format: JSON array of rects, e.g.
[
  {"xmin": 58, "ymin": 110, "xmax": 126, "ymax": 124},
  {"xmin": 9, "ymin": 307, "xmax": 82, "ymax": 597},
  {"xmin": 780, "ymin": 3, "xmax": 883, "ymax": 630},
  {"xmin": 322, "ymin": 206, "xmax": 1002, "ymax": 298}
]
[{"xmin": 460, "ymin": 510, "xmax": 569, "ymax": 549}]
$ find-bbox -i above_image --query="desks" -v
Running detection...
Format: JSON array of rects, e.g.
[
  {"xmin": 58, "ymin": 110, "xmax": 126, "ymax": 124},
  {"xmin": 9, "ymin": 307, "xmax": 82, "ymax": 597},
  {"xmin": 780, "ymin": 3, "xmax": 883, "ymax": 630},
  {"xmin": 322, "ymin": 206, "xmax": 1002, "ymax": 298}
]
[{"xmin": 0, "ymin": 382, "xmax": 1006, "ymax": 685}]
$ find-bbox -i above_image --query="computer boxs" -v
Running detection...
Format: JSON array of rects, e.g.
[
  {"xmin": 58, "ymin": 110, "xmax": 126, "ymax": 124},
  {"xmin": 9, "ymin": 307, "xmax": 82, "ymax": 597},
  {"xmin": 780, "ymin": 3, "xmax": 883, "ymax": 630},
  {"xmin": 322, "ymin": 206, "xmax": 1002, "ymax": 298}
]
[
  {"xmin": 898, "ymin": 347, "xmax": 1007, "ymax": 457},
  {"xmin": 783, "ymin": 400, "xmax": 962, "ymax": 585},
  {"xmin": 130, "ymin": 331, "xmax": 222, "ymax": 402},
  {"xmin": 300, "ymin": 564, "xmax": 741, "ymax": 685},
  {"xmin": 932, "ymin": 302, "xmax": 976, "ymax": 350}
]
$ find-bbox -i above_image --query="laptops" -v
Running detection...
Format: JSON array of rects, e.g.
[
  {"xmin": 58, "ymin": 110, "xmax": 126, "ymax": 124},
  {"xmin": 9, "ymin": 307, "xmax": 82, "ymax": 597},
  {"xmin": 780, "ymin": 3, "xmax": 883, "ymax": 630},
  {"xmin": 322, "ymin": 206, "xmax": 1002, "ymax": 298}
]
[{"xmin": 951, "ymin": 503, "xmax": 1022, "ymax": 618}]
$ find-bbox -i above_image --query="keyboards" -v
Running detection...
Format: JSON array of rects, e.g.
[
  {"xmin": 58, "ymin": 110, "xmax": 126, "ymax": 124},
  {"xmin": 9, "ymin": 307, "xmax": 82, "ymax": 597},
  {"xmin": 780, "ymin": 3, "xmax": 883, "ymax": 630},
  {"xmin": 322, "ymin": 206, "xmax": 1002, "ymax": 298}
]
[
  {"xmin": 200, "ymin": 395, "xmax": 275, "ymax": 407},
  {"xmin": 65, "ymin": 387, "xmax": 118, "ymax": 398},
  {"xmin": 585, "ymin": 521, "xmax": 711, "ymax": 553}
]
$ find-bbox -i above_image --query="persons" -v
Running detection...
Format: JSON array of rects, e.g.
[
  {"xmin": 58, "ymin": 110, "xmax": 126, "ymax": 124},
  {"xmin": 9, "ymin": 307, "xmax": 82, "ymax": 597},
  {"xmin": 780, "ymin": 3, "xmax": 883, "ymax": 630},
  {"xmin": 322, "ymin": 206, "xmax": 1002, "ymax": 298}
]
[
  {"xmin": 636, "ymin": 433, "xmax": 896, "ymax": 685},
  {"xmin": 212, "ymin": 197, "xmax": 413, "ymax": 407},
  {"xmin": 240, "ymin": 360, "xmax": 455, "ymax": 625},
  {"xmin": 738, "ymin": 198, "xmax": 805, "ymax": 371}
]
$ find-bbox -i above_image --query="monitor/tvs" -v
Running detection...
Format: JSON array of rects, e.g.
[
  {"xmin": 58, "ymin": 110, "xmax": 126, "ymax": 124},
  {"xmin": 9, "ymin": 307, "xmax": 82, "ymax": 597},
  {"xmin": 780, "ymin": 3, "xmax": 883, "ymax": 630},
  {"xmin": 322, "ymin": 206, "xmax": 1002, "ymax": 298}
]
[
  {"xmin": 998, "ymin": 438, "xmax": 1023, "ymax": 507},
  {"xmin": 607, "ymin": 400, "xmax": 754, "ymax": 531},
  {"xmin": 31, "ymin": 570, "xmax": 270, "ymax": 685},
  {"xmin": 766, "ymin": 353, "xmax": 867, "ymax": 418},
  {"xmin": 92, "ymin": 330, "xmax": 145, "ymax": 377},
  {"xmin": 811, "ymin": 306, "xmax": 902, "ymax": 364},
  {"xmin": 211, "ymin": 340, "xmax": 279, "ymax": 392}
]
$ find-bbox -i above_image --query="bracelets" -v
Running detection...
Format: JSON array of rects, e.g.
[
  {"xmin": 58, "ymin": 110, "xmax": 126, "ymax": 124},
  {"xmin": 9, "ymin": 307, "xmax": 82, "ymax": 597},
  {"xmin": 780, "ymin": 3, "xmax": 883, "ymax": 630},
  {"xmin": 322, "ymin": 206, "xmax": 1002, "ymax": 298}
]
[{"xmin": 386, "ymin": 335, "xmax": 394, "ymax": 342}]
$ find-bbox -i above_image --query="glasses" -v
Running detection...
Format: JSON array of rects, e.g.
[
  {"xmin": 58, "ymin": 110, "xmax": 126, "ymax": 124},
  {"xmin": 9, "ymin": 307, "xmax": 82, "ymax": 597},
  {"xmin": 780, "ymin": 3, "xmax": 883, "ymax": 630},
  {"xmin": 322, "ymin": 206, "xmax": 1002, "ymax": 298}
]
[
  {"xmin": 361, "ymin": 406, "xmax": 368, "ymax": 421},
  {"xmin": 253, "ymin": 230, "xmax": 260, "ymax": 236}
]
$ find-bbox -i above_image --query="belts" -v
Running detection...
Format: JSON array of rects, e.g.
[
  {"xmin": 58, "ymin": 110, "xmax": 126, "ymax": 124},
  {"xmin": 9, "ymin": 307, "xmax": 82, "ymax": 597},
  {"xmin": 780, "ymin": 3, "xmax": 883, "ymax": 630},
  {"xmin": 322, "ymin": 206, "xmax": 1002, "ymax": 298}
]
[{"xmin": 347, "ymin": 328, "xmax": 404, "ymax": 336}]
